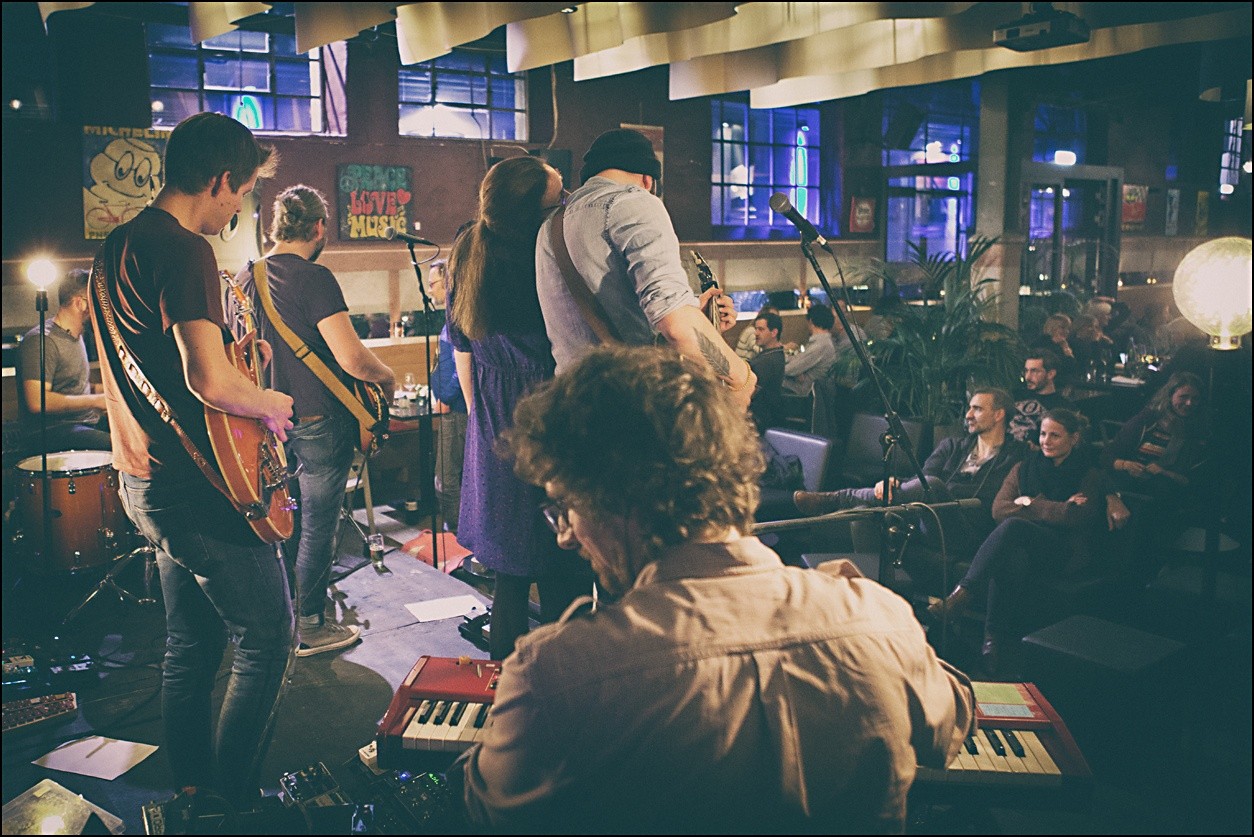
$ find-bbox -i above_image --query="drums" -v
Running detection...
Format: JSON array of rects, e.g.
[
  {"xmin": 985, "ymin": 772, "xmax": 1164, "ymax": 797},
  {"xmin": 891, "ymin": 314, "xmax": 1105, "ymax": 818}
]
[{"xmin": 13, "ymin": 447, "xmax": 125, "ymax": 577}]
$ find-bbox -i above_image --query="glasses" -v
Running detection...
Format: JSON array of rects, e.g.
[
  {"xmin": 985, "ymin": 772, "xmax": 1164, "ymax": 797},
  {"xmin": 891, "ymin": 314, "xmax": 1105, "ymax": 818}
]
[
  {"xmin": 543, "ymin": 187, "xmax": 567, "ymax": 211},
  {"xmin": 539, "ymin": 491, "xmax": 578, "ymax": 534}
]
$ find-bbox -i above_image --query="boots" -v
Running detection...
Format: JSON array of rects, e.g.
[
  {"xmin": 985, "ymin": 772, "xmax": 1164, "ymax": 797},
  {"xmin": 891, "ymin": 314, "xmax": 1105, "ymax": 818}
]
[{"xmin": 927, "ymin": 585, "xmax": 967, "ymax": 625}]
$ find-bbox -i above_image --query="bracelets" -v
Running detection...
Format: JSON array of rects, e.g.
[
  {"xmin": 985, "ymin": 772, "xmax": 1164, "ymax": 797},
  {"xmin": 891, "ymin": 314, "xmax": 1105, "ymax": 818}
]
[{"xmin": 726, "ymin": 360, "xmax": 751, "ymax": 393}]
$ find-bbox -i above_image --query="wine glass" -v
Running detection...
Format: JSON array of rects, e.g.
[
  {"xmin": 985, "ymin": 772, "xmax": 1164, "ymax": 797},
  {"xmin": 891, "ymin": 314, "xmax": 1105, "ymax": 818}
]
[
  {"xmin": 403, "ymin": 373, "xmax": 416, "ymax": 405},
  {"xmin": 391, "ymin": 383, "xmax": 404, "ymax": 408},
  {"xmin": 1136, "ymin": 344, "xmax": 1147, "ymax": 363}
]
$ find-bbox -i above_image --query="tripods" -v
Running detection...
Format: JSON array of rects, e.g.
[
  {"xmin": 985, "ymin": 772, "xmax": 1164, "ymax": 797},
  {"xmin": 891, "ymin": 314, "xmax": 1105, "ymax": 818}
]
[{"xmin": 54, "ymin": 542, "xmax": 156, "ymax": 633}]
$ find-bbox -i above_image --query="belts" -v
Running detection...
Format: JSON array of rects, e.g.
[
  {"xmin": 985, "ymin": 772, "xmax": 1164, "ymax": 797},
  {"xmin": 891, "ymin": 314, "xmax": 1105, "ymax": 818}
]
[{"xmin": 298, "ymin": 416, "xmax": 324, "ymax": 423}]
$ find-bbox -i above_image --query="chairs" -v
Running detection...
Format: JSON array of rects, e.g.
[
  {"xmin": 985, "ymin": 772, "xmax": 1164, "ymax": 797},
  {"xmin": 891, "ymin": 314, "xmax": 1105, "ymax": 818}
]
[{"xmin": 750, "ymin": 362, "xmax": 1213, "ymax": 654}]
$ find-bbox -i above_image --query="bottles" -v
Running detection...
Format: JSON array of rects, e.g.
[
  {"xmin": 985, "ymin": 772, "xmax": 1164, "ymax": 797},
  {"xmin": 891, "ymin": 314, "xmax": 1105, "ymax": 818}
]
[
  {"xmin": 393, "ymin": 321, "xmax": 406, "ymax": 339},
  {"xmin": 1126, "ymin": 337, "xmax": 1137, "ymax": 362},
  {"xmin": 368, "ymin": 533, "xmax": 384, "ymax": 571},
  {"xmin": 405, "ymin": 492, "xmax": 419, "ymax": 525}
]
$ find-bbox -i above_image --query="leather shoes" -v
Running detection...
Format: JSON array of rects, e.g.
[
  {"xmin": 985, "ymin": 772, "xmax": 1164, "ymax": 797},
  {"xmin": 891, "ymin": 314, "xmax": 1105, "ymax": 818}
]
[{"xmin": 793, "ymin": 490, "xmax": 840, "ymax": 517}]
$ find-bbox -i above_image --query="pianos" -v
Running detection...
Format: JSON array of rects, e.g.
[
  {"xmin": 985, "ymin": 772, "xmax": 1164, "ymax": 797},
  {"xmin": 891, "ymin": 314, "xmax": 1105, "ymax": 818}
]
[{"xmin": 376, "ymin": 651, "xmax": 503, "ymax": 774}]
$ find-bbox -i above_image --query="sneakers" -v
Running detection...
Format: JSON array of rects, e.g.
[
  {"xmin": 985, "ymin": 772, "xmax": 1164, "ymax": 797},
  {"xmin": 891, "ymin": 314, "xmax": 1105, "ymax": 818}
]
[{"xmin": 295, "ymin": 623, "xmax": 360, "ymax": 657}]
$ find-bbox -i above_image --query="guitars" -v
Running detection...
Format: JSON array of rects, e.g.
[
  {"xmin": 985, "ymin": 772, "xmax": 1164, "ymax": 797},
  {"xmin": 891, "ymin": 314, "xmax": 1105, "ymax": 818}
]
[
  {"xmin": 678, "ymin": 245, "xmax": 767, "ymax": 481},
  {"xmin": 198, "ymin": 267, "xmax": 299, "ymax": 544},
  {"xmin": 348, "ymin": 378, "xmax": 391, "ymax": 459}
]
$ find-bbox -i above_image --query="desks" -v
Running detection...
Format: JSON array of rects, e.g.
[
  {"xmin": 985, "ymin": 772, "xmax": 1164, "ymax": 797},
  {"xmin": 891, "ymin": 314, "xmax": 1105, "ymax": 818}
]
[
  {"xmin": 390, "ymin": 399, "xmax": 451, "ymax": 524},
  {"xmin": 1057, "ymin": 384, "xmax": 1113, "ymax": 408},
  {"xmin": 1106, "ymin": 358, "xmax": 1169, "ymax": 393}
]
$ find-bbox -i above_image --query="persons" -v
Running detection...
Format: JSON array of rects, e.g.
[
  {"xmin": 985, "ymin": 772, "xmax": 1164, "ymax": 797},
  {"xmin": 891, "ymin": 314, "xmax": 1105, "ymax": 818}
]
[
  {"xmin": 223, "ymin": 185, "xmax": 396, "ymax": 659},
  {"xmin": 455, "ymin": 346, "xmax": 977, "ymax": 837},
  {"xmin": 428, "ymin": 259, "xmax": 468, "ymax": 536},
  {"xmin": 448, "ymin": 156, "xmax": 594, "ymax": 660},
  {"xmin": 17, "ymin": 270, "xmax": 113, "ymax": 452},
  {"xmin": 536, "ymin": 129, "xmax": 760, "ymax": 411},
  {"xmin": 87, "ymin": 112, "xmax": 296, "ymax": 806},
  {"xmin": 733, "ymin": 290, "xmax": 1207, "ymax": 679}
]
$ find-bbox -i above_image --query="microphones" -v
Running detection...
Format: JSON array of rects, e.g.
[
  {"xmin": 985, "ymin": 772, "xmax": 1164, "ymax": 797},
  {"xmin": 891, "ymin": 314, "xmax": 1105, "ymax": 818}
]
[
  {"xmin": 384, "ymin": 226, "xmax": 434, "ymax": 246},
  {"xmin": 769, "ymin": 192, "xmax": 835, "ymax": 255}
]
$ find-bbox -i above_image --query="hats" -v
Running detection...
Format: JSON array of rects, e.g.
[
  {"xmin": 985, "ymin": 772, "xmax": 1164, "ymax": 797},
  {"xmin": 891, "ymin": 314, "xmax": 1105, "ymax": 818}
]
[{"xmin": 581, "ymin": 128, "xmax": 661, "ymax": 186}]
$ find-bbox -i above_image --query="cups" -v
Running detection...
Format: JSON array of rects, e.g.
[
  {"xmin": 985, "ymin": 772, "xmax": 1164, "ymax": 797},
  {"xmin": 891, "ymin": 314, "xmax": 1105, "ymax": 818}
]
[{"xmin": 1148, "ymin": 346, "xmax": 1159, "ymax": 366}]
